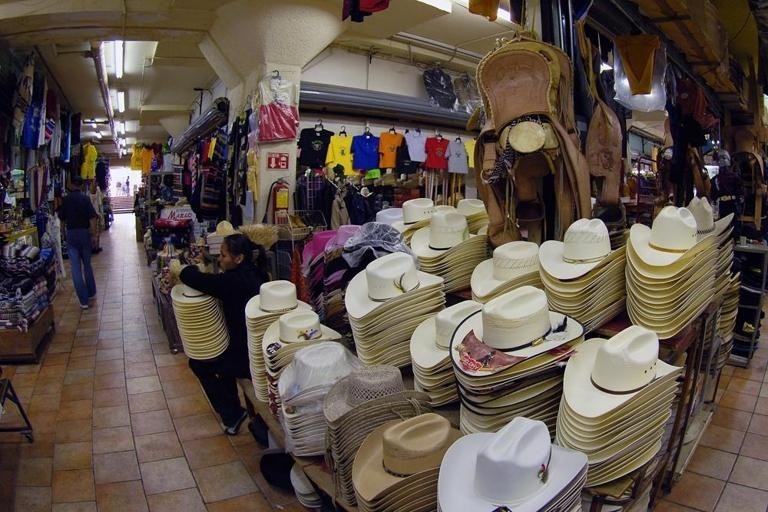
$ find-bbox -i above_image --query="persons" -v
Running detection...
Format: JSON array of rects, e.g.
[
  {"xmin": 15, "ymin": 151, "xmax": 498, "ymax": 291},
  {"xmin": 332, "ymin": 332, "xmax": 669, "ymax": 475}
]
[
  {"xmin": 710, "ymin": 149, "xmax": 745, "ymax": 245},
  {"xmin": 175, "ymin": 233, "xmax": 271, "ymax": 437},
  {"xmin": 58, "ymin": 176, "xmax": 99, "ymax": 311}
]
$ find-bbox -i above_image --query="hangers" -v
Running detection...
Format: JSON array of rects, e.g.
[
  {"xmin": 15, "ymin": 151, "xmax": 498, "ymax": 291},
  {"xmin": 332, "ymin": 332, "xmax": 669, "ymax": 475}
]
[
  {"xmin": 302, "ymin": 115, "xmax": 479, "ymax": 142},
  {"xmin": 270, "ymin": 68, "xmax": 282, "ymax": 81}
]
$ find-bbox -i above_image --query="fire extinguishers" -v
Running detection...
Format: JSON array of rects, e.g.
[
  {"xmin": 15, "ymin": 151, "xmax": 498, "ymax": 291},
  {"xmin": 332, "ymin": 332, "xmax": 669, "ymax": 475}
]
[{"xmin": 263, "ymin": 175, "xmax": 290, "ymax": 224}]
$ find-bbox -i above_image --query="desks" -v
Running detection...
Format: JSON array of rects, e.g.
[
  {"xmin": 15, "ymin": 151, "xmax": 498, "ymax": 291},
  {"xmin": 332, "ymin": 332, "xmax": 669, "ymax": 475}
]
[{"xmin": 0, "ymin": 303, "xmax": 56, "ymax": 364}]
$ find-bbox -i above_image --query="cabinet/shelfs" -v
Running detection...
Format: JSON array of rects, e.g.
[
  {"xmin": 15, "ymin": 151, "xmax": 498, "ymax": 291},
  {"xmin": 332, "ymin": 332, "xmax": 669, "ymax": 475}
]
[{"xmin": 628, "ymin": 154, "xmax": 657, "ymax": 226}]
